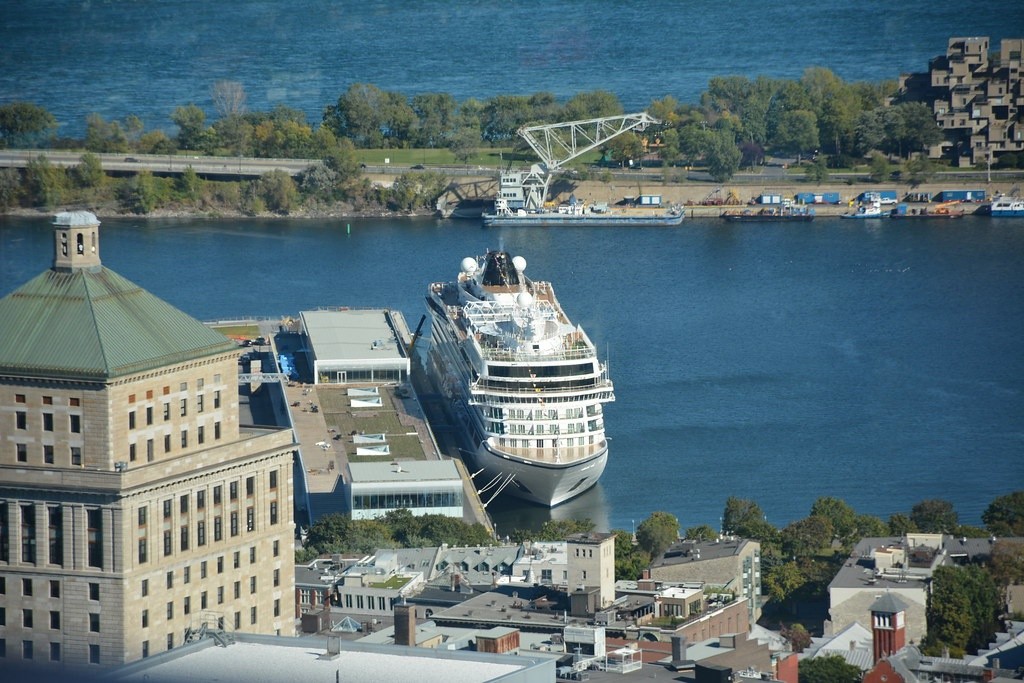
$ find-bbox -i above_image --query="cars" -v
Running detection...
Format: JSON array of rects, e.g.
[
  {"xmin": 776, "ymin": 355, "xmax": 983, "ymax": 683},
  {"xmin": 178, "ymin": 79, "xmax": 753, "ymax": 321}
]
[
  {"xmin": 410, "ymin": 165, "xmax": 423, "ymax": 169},
  {"xmin": 124, "ymin": 157, "xmax": 140, "ymax": 163}
]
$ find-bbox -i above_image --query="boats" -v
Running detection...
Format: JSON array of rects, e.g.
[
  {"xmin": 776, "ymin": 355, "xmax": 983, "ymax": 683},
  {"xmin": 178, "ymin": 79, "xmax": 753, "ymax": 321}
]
[
  {"xmin": 841, "ymin": 202, "xmax": 899, "ymax": 218},
  {"xmin": 719, "ymin": 202, "xmax": 815, "ymax": 222},
  {"xmin": 423, "ymin": 248, "xmax": 615, "ymax": 509},
  {"xmin": 991, "ymin": 197, "xmax": 1024, "ymax": 217},
  {"xmin": 481, "ymin": 149, "xmax": 686, "ymax": 226}
]
